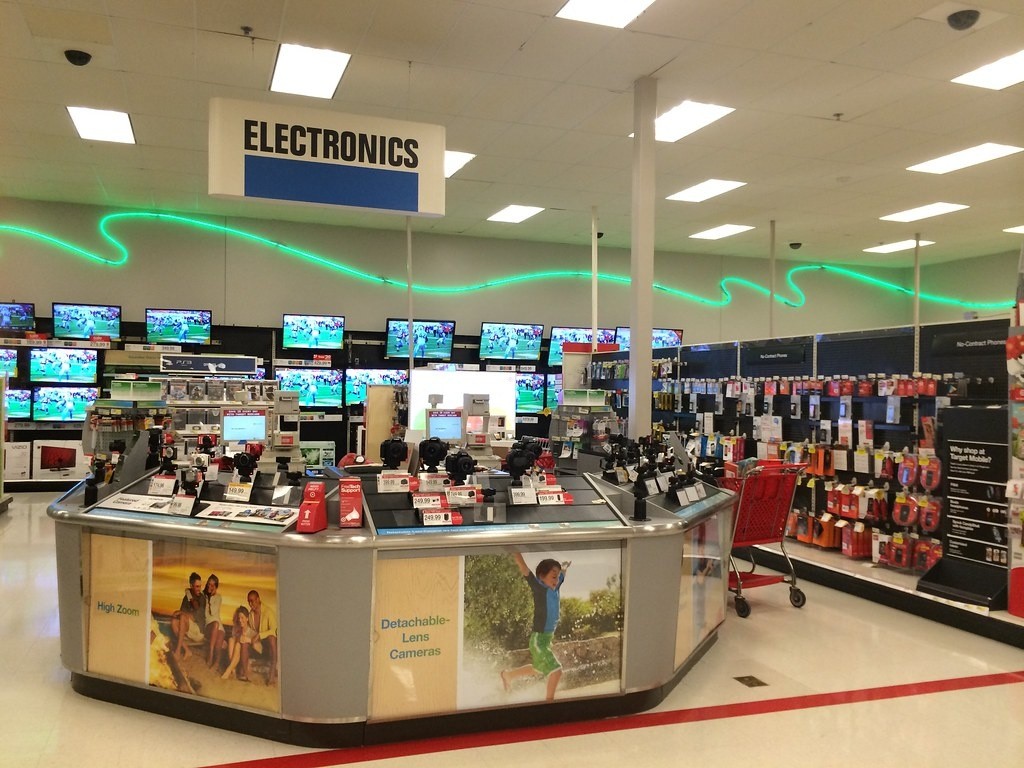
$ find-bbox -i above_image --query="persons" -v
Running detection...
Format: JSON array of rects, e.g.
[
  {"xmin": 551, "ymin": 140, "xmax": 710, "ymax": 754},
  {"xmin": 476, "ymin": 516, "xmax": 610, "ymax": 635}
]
[
  {"xmin": 150, "ymin": 312, "xmax": 210, "ymax": 343},
  {"xmin": 285, "ymin": 318, "xmax": 342, "ymax": 347},
  {"xmin": 553, "ymin": 331, "xmax": 614, "ymax": 359},
  {"xmin": 347, "ymin": 371, "xmax": 407, "ymax": 398},
  {"xmin": 36, "ymin": 389, "xmax": 96, "ymax": 419},
  {"xmin": 515, "ymin": 376, "xmax": 542, "ymax": 410},
  {"xmin": 513, "ymin": 551, "xmax": 573, "ymax": 700},
  {"xmin": 0, "ymin": 351, "xmax": 17, "ymax": 370},
  {"xmin": 33, "ymin": 351, "xmax": 94, "ymax": 381},
  {"xmin": 0, "ymin": 304, "xmax": 36, "ymax": 333},
  {"xmin": 172, "ymin": 571, "xmax": 277, "ymax": 685},
  {"xmin": 5, "ymin": 391, "xmax": 30, "ymax": 411},
  {"xmin": 390, "ymin": 322, "xmax": 451, "ymax": 358},
  {"xmin": 484, "ymin": 326, "xmax": 542, "ymax": 360},
  {"xmin": 56, "ymin": 308, "xmax": 118, "ymax": 337},
  {"xmin": 653, "ymin": 331, "xmax": 679, "ymax": 348},
  {"xmin": 277, "ymin": 370, "xmax": 343, "ymax": 406}
]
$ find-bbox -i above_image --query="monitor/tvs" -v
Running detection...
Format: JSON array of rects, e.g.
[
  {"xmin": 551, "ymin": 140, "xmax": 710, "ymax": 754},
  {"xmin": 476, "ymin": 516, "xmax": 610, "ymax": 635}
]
[
  {"xmin": 40, "ymin": 446, "xmax": 76, "ymax": 471},
  {"xmin": 0, "ymin": 301, "xmax": 684, "ymax": 447}
]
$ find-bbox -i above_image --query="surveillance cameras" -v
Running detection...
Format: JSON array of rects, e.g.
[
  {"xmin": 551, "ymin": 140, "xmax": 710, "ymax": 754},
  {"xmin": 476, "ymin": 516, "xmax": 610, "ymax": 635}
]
[
  {"xmin": 597, "ymin": 232, "xmax": 604, "ymax": 238},
  {"xmin": 947, "ymin": 10, "xmax": 980, "ymax": 30},
  {"xmin": 790, "ymin": 243, "xmax": 802, "ymax": 250},
  {"xmin": 64, "ymin": 50, "xmax": 92, "ymax": 66}
]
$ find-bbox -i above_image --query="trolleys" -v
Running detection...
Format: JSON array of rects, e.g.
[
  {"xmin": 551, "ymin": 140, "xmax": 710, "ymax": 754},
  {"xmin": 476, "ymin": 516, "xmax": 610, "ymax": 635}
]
[{"xmin": 694, "ymin": 458, "xmax": 812, "ymax": 618}]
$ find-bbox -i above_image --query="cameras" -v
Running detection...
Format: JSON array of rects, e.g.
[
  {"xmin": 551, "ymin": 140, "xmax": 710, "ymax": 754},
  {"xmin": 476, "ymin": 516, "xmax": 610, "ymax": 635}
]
[
  {"xmin": 602, "ymin": 427, "xmax": 696, "ymax": 493},
  {"xmin": 379, "ymin": 436, "xmax": 543, "ymax": 484},
  {"xmin": 108, "ymin": 428, "xmax": 264, "ymax": 487}
]
